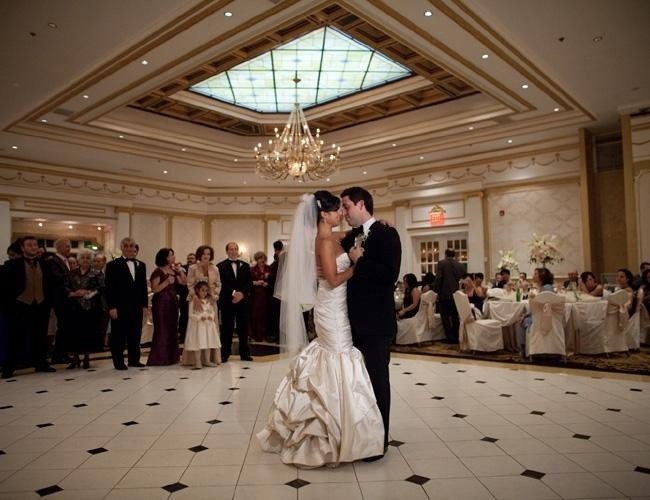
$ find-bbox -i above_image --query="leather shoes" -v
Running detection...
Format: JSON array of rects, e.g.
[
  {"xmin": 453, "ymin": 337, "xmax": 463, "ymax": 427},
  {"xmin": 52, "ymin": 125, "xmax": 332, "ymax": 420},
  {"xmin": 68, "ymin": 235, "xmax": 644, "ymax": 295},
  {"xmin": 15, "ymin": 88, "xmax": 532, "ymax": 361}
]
[
  {"xmin": 114, "ymin": 363, "xmax": 128, "ymax": 371},
  {"xmin": 35, "ymin": 366, "xmax": 56, "ymax": 373},
  {"xmin": 127, "ymin": 361, "xmax": 146, "ymax": 368}
]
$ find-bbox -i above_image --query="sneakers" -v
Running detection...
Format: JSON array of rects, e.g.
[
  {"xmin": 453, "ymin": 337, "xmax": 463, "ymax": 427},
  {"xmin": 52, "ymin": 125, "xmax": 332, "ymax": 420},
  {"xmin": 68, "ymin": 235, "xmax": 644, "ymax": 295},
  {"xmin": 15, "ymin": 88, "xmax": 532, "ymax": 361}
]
[
  {"xmin": 194, "ymin": 361, "xmax": 203, "ymax": 369},
  {"xmin": 204, "ymin": 360, "xmax": 217, "ymax": 367}
]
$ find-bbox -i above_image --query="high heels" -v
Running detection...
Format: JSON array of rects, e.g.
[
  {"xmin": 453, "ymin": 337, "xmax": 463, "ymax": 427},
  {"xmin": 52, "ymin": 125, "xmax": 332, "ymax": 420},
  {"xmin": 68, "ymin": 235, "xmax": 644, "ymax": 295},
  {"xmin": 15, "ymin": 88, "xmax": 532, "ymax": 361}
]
[
  {"xmin": 82, "ymin": 359, "xmax": 90, "ymax": 369},
  {"xmin": 66, "ymin": 359, "xmax": 81, "ymax": 370}
]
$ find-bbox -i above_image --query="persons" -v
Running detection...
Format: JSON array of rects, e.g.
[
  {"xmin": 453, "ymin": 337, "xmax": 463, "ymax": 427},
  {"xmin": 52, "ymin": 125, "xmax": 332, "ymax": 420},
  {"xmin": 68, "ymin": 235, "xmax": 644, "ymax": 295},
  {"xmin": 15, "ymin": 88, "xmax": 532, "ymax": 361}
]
[
  {"xmin": 398, "ymin": 247, "xmax": 650, "ymax": 358},
  {"xmin": 256, "ymin": 190, "xmax": 396, "ymax": 469},
  {"xmin": 0, "ymin": 237, "xmax": 315, "ymax": 378},
  {"xmin": 315, "ymin": 186, "xmax": 402, "ymax": 463}
]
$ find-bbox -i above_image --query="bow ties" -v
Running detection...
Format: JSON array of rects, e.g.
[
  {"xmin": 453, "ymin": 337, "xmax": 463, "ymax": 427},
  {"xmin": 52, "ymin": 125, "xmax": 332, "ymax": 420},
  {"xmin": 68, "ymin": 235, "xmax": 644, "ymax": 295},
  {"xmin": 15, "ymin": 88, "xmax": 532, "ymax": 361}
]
[
  {"xmin": 228, "ymin": 259, "xmax": 237, "ymax": 263},
  {"xmin": 351, "ymin": 225, "xmax": 365, "ymax": 237},
  {"xmin": 125, "ymin": 258, "xmax": 135, "ymax": 262}
]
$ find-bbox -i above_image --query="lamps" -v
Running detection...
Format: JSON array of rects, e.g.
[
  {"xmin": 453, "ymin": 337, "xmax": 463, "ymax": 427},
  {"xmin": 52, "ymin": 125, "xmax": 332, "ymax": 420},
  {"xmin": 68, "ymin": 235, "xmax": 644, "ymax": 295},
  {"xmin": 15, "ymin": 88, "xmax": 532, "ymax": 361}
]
[
  {"xmin": 251, "ymin": 68, "xmax": 342, "ymax": 186},
  {"xmin": 427, "ymin": 205, "xmax": 447, "ymax": 226}
]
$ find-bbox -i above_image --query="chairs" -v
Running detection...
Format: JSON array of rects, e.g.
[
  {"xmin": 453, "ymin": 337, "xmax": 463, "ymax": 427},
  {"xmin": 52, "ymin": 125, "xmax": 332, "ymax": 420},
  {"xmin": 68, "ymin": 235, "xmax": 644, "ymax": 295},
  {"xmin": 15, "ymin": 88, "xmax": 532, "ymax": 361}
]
[
  {"xmin": 632, "ymin": 287, "xmax": 645, "ymax": 352},
  {"xmin": 453, "ymin": 291, "xmax": 505, "ymax": 355},
  {"xmin": 580, "ymin": 290, "xmax": 630, "ymax": 356},
  {"xmin": 395, "ymin": 289, "xmax": 437, "ymax": 345},
  {"xmin": 523, "ymin": 291, "xmax": 568, "ymax": 365}
]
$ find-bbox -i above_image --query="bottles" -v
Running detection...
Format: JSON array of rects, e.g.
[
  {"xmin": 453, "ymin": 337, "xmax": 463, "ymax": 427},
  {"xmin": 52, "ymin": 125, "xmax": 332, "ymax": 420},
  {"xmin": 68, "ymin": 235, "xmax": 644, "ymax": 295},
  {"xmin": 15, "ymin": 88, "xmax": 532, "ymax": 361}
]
[{"xmin": 516, "ymin": 287, "xmax": 520, "ymax": 302}]
